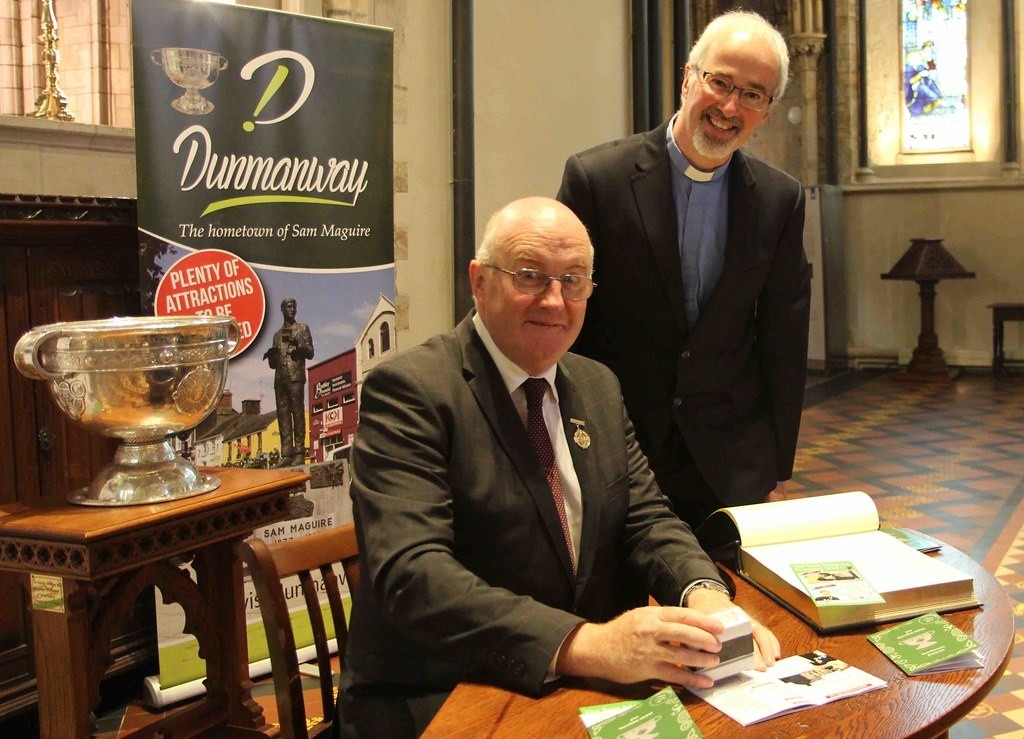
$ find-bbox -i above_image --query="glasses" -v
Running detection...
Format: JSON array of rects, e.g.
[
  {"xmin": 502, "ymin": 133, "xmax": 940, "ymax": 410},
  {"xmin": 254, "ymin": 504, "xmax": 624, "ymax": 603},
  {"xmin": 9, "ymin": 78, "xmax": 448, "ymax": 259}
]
[
  {"xmin": 690, "ymin": 65, "xmax": 775, "ymax": 113},
  {"xmin": 483, "ymin": 261, "xmax": 597, "ymax": 301}
]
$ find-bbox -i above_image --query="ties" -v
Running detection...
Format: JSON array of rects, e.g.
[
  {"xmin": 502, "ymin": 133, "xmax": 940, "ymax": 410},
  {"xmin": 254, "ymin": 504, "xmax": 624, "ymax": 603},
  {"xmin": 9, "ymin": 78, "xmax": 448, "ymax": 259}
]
[{"xmin": 520, "ymin": 378, "xmax": 577, "ymax": 579}]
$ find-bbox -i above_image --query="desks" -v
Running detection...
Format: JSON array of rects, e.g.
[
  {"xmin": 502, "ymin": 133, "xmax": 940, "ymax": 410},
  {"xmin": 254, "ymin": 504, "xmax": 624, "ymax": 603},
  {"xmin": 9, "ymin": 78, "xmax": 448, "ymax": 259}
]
[
  {"xmin": 415, "ymin": 530, "xmax": 1017, "ymax": 739},
  {"xmin": 0, "ymin": 468, "xmax": 312, "ymax": 739}
]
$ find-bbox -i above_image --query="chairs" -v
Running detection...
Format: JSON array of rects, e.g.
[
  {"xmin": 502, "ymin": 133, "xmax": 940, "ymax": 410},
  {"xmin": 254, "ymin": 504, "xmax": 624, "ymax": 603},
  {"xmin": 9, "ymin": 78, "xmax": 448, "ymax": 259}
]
[{"xmin": 242, "ymin": 521, "xmax": 359, "ymax": 739}]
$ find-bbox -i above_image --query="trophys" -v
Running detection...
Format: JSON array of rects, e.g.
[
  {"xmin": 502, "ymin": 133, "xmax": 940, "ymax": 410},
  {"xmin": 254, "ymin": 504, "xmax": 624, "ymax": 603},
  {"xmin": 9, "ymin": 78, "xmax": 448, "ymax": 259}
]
[
  {"xmin": 11, "ymin": 314, "xmax": 241, "ymax": 507},
  {"xmin": 149, "ymin": 47, "xmax": 228, "ymax": 115}
]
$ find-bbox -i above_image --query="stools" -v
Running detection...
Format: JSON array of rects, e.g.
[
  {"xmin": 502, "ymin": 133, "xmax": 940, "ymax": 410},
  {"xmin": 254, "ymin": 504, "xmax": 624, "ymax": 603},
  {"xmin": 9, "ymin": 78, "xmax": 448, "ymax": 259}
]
[{"xmin": 985, "ymin": 303, "xmax": 1024, "ymax": 378}]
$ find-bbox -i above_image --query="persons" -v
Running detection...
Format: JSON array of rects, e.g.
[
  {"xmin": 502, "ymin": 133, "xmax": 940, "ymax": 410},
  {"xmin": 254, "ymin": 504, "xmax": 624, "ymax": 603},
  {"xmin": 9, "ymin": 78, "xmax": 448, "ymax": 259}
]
[
  {"xmin": 266, "ymin": 298, "xmax": 314, "ymax": 467},
  {"xmin": 554, "ymin": 11, "xmax": 812, "ymax": 549},
  {"xmin": 339, "ymin": 196, "xmax": 780, "ymax": 739}
]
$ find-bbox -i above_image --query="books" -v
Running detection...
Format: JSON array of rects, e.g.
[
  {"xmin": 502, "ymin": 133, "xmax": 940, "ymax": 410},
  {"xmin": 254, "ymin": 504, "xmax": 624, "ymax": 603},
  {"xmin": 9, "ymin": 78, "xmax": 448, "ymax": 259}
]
[
  {"xmin": 586, "ymin": 686, "xmax": 702, "ymax": 739},
  {"xmin": 686, "ymin": 650, "xmax": 888, "ymax": 727},
  {"xmin": 695, "ymin": 491, "xmax": 985, "ymax": 632},
  {"xmin": 881, "ymin": 528, "xmax": 943, "ymax": 553},
  {"xmin": 866, "ymin": 612, "xmax": 984, "ymax": 676}
]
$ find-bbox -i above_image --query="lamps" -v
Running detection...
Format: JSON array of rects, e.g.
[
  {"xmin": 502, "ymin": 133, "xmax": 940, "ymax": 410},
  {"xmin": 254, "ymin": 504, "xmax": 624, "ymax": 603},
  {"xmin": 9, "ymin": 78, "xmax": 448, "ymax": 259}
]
[{"xmin": 880, "ymin": 238, "xmax": 976, "ymax": 382}]
